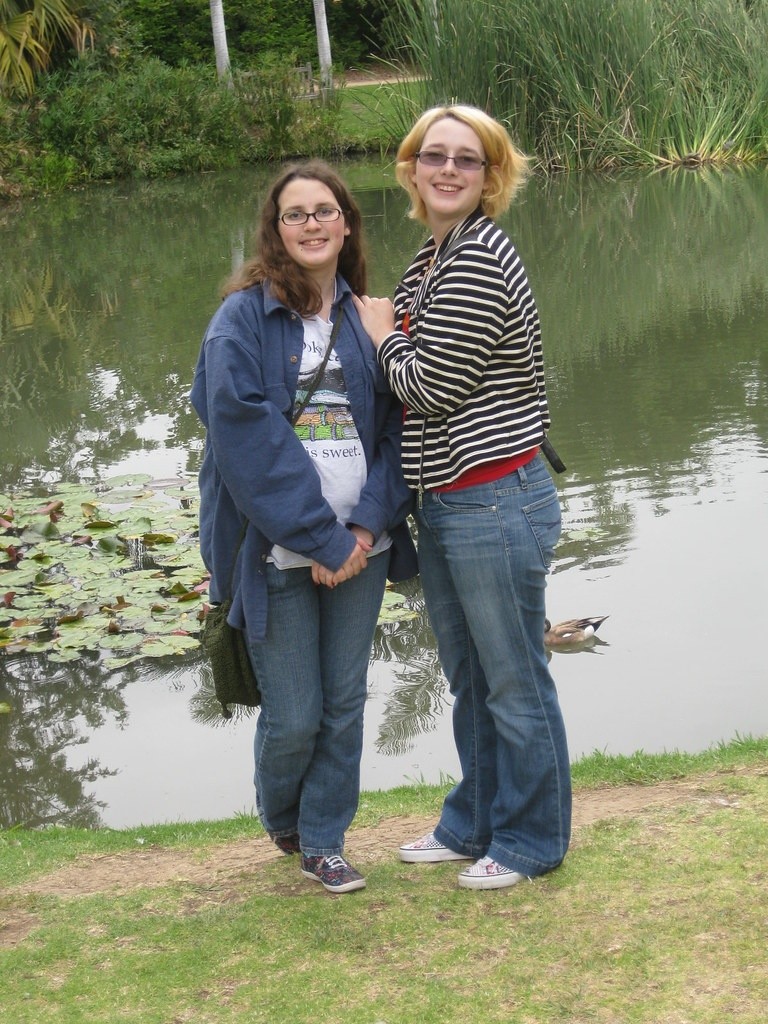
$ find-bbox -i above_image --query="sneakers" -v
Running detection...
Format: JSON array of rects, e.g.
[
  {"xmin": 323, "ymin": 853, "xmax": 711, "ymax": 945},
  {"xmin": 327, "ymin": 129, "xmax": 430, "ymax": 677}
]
[
  {"xmin": 300, "ymin": 852, "xmax": 365, "ymax": 893},
  {"xmin": 400, "ymin": 830, "xmax": 478, "ymax": 862},
  {"xmin": 456, "ymin": 855, "xmax": 525, "ymax": 890},
  {"xmin": 268, "ymin": 830, "xmax": 302, "ymax": 855}
]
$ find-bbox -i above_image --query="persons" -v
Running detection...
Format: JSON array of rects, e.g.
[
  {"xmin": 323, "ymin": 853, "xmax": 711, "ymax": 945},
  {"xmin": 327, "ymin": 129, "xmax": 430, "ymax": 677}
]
[
  {"xmin": 189, "ymin": 164, "xmax": 419, "ymax": 893},
  {"xmin": 351, "ymin": 105, "xmax": 572, "ymax": 889}
]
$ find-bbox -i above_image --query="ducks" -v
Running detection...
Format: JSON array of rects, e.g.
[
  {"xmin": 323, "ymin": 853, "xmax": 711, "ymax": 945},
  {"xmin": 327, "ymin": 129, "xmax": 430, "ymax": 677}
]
[
  {"xmin": 544, "ymin": 616, "xmax": 609, "ymax": 644},
  {"xmin": 545, "ymin": 636, "xmax": 610, "ymax": 664}
]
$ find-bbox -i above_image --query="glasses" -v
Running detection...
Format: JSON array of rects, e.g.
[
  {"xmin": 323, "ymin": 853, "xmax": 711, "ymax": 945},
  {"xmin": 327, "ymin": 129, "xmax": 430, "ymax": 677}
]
[
  {"xmin": 415, "ymin": 150, "xmax": 486, "ymax": 170},
  {"xmin": 274, "ymin": 208, "xmax": 344, "ymax": 226}
]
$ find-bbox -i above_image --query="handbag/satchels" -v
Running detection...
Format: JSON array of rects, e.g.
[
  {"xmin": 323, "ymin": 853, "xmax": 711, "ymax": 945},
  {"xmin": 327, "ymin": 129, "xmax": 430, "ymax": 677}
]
[
  {"xmin": 203, "ymin": 600, "xmax": 262, "ymax": 719},
  {"xmin": 387, "ymin": 491, "xmax": 420, "ymax": 583}
]
